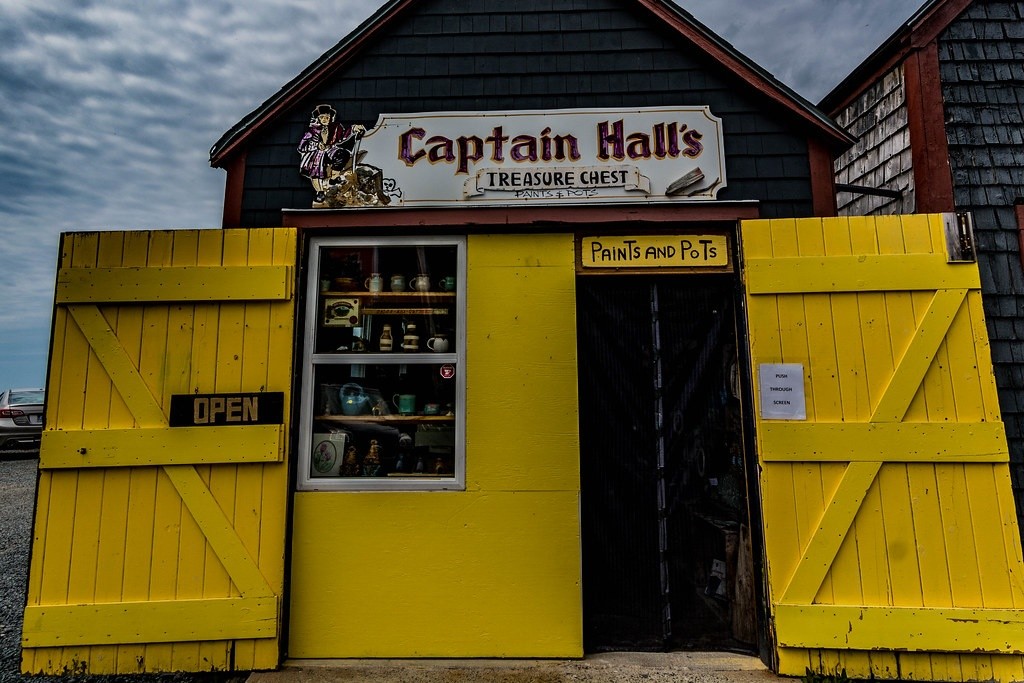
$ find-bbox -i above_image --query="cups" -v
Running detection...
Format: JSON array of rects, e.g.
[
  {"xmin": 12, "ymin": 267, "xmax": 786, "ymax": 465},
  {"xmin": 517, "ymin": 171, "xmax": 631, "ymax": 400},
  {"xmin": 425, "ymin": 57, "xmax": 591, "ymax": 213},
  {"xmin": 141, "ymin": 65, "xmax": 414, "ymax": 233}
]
[
  {"xmin": 427, "ymin": 334, "xmax": 448, "ymax": 352},
  {"xmin": 439, "ymin": 277, "xmax": 457, "ymax": 292},
  {"xmin": 391, "ymin": 276, "xmax": 405, "ymax": 292},
  {"xmin": 409, "ymin": 274, "xmax": 430, "ymax": 291},
  {"xmin": 393, "ymin": 394, "xmax": 416, "ymax": 415},
  {"xmin": 364, "ymin": 272, "xmax": 384, "ymax": 292},
  {"xmin": 425, "ymin": 404, "xmax": 439, "ymax": 414}
]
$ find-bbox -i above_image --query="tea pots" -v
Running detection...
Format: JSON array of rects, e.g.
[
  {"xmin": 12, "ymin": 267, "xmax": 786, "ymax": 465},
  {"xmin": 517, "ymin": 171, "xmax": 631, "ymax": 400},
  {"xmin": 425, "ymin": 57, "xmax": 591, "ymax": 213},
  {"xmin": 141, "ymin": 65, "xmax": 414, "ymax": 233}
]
[{"xmin": 340, "ymin": 383, "xmax": 370, "ymax": 416}]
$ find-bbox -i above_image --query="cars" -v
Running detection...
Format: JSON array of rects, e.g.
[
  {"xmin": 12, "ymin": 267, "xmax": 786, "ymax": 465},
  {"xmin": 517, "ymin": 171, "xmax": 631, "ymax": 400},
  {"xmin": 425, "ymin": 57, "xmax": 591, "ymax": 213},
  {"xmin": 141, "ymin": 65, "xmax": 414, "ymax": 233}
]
[{"xmin": 0, "ymin": 388, "xmax": 46, "ymax": 448}]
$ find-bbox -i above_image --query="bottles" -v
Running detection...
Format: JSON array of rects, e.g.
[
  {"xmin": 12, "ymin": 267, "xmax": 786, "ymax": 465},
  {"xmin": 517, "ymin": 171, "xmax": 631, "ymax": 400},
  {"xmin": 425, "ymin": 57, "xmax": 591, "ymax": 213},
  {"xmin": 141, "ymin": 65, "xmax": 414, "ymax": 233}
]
[
  {"xmin": 404, "ymin": 321, "xmax": 419, "ymax": 351},
  {"xmin": 435, "ymin": 458, "xmax": 445, "ymax": 474},
  {"xmin": 416, "ymin": 457, "xmax": 423, "ymax": 473},
  {"xmin": 396, "ymin": 456, "xmax": 403, "ymax": 470},
  {"xmin": 416, "ymin": 424, "xmax": 439, "ymax": 432},
  {"xmin": 380, "ymin": 324, "xmax": 393, "ymax": 352}
]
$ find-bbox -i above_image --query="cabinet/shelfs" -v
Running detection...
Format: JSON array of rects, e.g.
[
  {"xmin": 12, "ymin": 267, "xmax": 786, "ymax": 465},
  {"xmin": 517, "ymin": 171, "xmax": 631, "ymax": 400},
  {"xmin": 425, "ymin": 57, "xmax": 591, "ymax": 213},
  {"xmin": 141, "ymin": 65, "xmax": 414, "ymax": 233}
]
[{"xmin": 318, "ymin": 287, "xmax": 454, "ymax": 478}]
